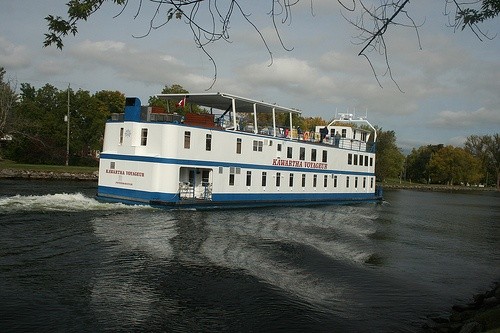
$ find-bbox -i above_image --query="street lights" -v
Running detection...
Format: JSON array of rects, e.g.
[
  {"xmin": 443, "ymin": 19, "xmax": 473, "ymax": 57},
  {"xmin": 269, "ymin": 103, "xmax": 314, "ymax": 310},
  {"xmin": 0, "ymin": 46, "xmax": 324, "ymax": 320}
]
[{"xmin": 65, "ymin": 82, "xmax": 72, "ymax": 166}]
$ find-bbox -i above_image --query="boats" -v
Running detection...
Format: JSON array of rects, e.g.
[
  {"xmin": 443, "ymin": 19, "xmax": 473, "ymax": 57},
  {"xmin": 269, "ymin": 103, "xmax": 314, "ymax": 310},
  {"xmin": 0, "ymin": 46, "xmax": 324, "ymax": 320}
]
[{"xmin": 95, "ymin": 93, "xmax": 384, "ymax": 210}]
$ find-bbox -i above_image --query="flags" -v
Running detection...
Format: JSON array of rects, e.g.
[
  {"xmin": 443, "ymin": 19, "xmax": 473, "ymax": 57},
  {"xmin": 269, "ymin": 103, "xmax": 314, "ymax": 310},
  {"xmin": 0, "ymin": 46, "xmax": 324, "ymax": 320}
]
[{"xmin": 175, "ymin": 96, "xmax": 185, "ymax": 108}]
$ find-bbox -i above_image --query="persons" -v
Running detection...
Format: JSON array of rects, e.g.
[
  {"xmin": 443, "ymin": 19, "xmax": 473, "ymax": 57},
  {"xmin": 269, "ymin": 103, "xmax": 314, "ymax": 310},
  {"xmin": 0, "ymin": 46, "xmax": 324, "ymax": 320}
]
[{"xmin": 285, "ymin": 125, "xmax": 342, "ymax": 147}]
